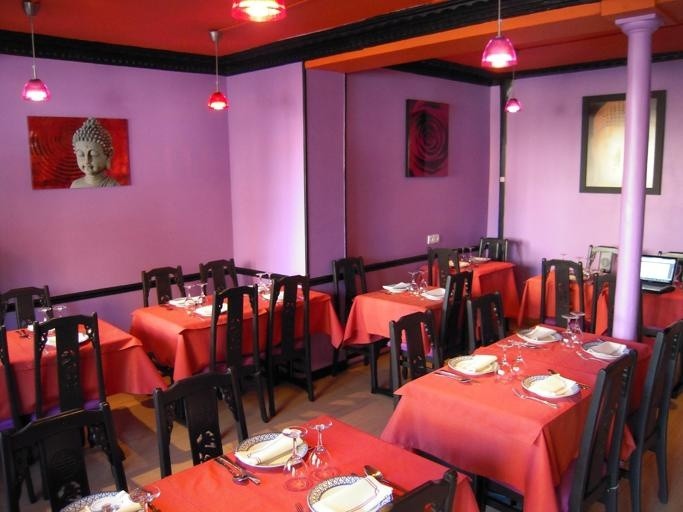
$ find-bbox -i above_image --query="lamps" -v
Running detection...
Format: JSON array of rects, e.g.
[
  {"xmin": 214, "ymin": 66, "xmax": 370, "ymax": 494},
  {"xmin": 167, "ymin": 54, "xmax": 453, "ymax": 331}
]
[
  {"xmin": 206, "ymin": 30, "xmax": 230, "ymax": 112},
  {"xmin": 227, "ymin": 2, "xmax": 288, "ymax": 24},
  {"xmin": 480, "ymin": 1, "xmax": 518, "ymax": 71},
  {"xmin": 17, "ymin": 0, "xmax": 51, "ymax": 105},
  {"xmin": 504, "ymin": 72, "xmax": 521, "ymax": 113}
]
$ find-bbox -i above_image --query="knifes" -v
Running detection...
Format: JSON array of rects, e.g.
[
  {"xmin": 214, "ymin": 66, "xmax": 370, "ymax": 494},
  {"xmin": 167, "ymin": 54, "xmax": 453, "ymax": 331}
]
[{"xmin": 219, "ymin": 454, "xmax": 260, "ymax": 484}]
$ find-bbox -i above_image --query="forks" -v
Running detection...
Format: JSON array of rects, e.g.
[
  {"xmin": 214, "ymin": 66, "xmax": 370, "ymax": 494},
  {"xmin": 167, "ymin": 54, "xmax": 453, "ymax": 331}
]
[{"xmin": 512, "ymin": 387, "xmax": 556, "ymax": 409}]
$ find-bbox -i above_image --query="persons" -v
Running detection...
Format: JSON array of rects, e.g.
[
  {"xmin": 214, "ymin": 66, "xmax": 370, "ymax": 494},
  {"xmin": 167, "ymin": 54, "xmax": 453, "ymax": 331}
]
[{"xmin": 65, "ymin": 118, "xmax": 121, "ymax": 188}]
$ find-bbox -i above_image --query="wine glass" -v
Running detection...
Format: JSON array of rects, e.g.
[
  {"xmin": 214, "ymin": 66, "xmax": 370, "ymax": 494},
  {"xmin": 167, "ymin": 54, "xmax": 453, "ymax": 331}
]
[
  {"xmin": 129, "ymin": 485, "xmax": 160, "ymax": 511},
  {"xmin": 569, "ymin": 311, "xmax": 586, "ymax": 343},
  {"xmin": 245, "ymin": 272, "xmax": 284, "ymax": 301},
  {"xmin": 167, "ymin": 281, "xmax": 227, "ymax": 317},
  {"xmin": 495, "ymin": 339, "xmax": 512, "ymax": 383},
  {"xmin": 385, "ymin": 269, "xmax": 451, "ymax": 300},
  {"xmin": 507, "ymin": 338, "xmax": 527, "ymax": 380},
  {"xmin": 446, "ymin": 247, "xmax": 491, "ymax": 268},
  {"xmin": 557, "ymin": 253, "xmax": 594, "ymax": 282},
  {"xmin": 279, "ymin": 424, "xmax": 315, "ymax": 491},
  {"xmin": 304, "ymin": 418, "xmax": 344, "ymax": 479},
  {"xmin": 16, "ymin": 304, "xmax": 66, "ymax": 339},
  {"xmin": 557, "ymin": 313, "xmax": 574, "ymax": 349}
]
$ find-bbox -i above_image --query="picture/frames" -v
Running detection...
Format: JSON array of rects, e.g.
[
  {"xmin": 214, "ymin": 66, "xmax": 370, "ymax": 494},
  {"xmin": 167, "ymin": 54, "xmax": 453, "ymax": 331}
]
[{"xmin": 578, "ymin": 88, "xmax": 667, "ymax": 194}]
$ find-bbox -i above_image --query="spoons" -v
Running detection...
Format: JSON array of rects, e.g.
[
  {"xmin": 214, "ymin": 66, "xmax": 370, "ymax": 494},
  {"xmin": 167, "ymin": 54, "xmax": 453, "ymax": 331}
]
[
  {"xmin": 214, "ymin": 458, "xmax": 247, "ymax": 482},
  {"xmin": 548, "ymin": 368, "xmax": 587, "ymax": 389},
  {"xmin": 362, "ymin": 464, "xmax": 408, "ymax": 493}
]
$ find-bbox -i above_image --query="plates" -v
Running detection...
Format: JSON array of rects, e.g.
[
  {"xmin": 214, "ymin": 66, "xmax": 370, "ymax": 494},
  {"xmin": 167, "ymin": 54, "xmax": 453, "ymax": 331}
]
[
  {"xmin": 58, "ymin": 490, "xmax": 142, "ymax": 511},
  {"xmin": 516, "ymin": 330, "xmax": 561, "ymax": 345},
  {"xmin": 77, "ymin": 331, "xmax": 88, "ymax": 344},
  {"xmin": 521, "ymin": 375, "xmax": 580, "ymax": 398},
  {"xmin": 448, "ymin": 355, "xmax": 497, "ymax": 375},
  {"xmin": 235, "ymin": 431, "xmax": 307, "ymax": 467},
  {"xmin": 306, "ymin": 474, "xmax": 393, "ymax": 512},
  {"xmin": 581, "ymin": 340, "xmax": 629, "ymax": 360}
]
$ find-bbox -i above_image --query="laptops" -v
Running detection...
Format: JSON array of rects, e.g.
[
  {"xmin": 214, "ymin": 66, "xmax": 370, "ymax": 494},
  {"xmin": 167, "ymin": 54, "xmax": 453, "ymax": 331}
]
[{"xmin": 640, "ymin": 255, "xmax": 677, "ymax": 293}]
